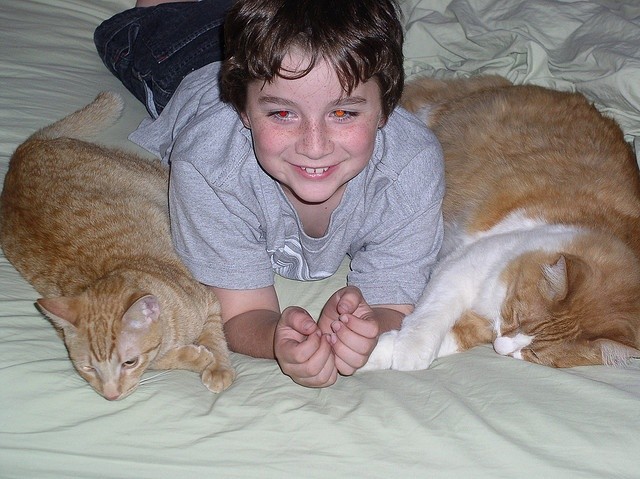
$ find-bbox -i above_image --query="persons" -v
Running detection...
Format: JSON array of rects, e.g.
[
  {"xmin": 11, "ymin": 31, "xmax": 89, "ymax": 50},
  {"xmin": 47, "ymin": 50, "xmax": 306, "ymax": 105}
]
[{"xmin": 93, "ymin": 0, "xmax": 446, "ymax": 389}]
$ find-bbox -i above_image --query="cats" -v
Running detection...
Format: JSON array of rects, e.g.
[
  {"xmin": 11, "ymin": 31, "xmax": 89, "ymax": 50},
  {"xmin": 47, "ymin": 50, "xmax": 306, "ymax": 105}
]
[
  {"xmin": 0, "ymin": 89, "xmax": 236, "ymax": 403},
  {"xmin": 356, "ymin": 75, "xmax": 640, "ymax": 372}
]
